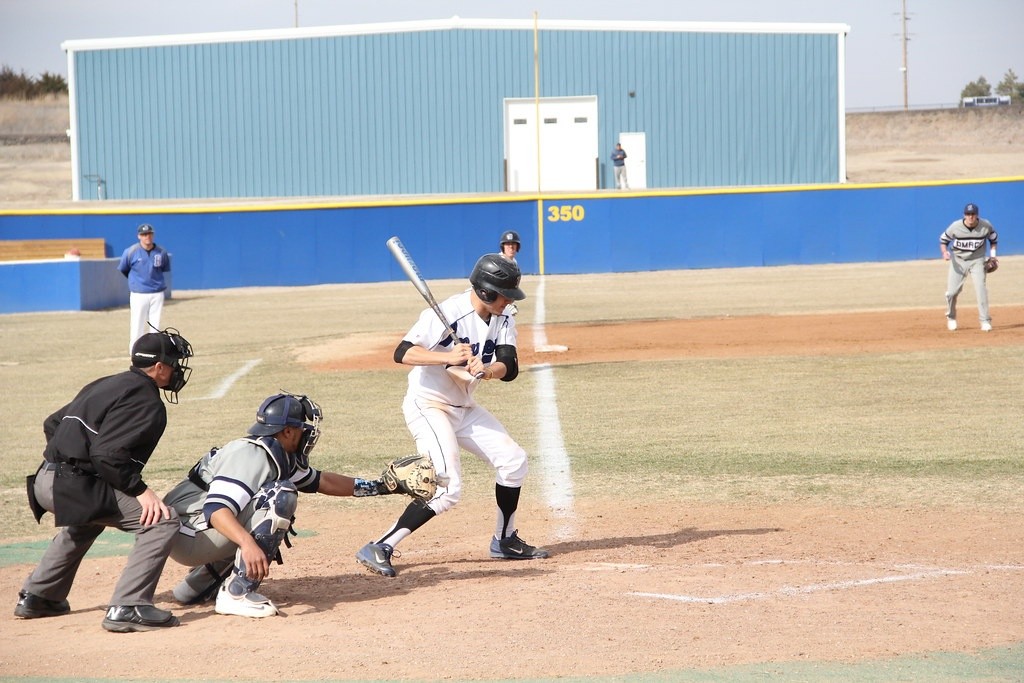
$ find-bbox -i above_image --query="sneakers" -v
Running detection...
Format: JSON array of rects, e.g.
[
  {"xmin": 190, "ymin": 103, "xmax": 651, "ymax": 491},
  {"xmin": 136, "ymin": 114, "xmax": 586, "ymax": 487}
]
[
  {"xmin": 355, "ymin": 542, "xmax": 401, "ymax": 577},
  {"xmin": 173, "ymin": 579, "xmax": 211, "ymax": 603},
  {"xmin": 215, "ymin": 586, "xmax": 275, "ymax": 618},
  {"xmin": 980, "ymin": 320, "xmax": 992, "ymax": 330},
  {"xmin": 14, "ymin": 590, "xmax": 71, "ymax": 618},
  {"xmin": 103, "ymin": 605, "xmax": 180, "ymax": 633},
  {"xmin": 490, "ymin": 529, "xmax": 548, "ymax": 560},
  {"xmin": 946, "ymin": 316, "xmax": 957, "ymax": 330}
]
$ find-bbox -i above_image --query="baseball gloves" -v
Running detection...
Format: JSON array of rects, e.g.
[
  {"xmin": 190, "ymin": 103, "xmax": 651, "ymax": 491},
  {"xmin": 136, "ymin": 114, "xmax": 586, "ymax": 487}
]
[
  {"xmin": 377, "ymin": 454, "xmax": 438, "ymax": 501},
  {"xmin": 984, "ymin": 257, "xmax": 999, "ymax": 273}
]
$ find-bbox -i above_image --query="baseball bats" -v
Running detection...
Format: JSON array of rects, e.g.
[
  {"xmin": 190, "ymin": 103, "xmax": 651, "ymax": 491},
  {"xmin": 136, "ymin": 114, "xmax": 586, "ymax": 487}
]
[{"xmin": 385, "ymin": 235, "xmax": 485, "ymax": 382}]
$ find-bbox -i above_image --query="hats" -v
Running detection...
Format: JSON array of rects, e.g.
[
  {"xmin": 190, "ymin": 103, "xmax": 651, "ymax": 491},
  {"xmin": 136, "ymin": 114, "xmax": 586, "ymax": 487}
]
[{"xmin": 131, "ymin": 333, "xmax": 162, "ymax": 368}]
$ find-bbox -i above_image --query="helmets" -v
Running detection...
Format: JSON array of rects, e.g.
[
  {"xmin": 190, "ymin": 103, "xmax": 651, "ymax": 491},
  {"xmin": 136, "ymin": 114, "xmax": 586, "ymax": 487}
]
[
  {"xmin": 964, "ymin": 203, "xmax": 978, "ymax": 215},
  {"xmin": 499, "ymin": 231, "xmax": 522, "ymax": 253},
  {"xmin": 470, "ymin": 253, "xmax": 526, "ymax": 305},
  {"xmin": 138, "ymin": 224, "xmax": 155, "ymax": 236},
  {"xmin": 247, "ymin": 394, "xmax": 323, "ymax": 472}
]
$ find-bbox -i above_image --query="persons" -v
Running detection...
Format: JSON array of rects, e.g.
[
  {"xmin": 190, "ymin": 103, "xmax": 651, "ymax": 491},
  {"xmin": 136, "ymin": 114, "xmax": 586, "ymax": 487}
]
[
  {"xmin": 14, "ymin": 320, "xmax": 193, "ymax": 633},
  {"xmin": 496, "ymin": 230, "xmax": 522, "ymax": 317},
  {"xmin": 162, "ymin": 389, "xmax": 451, "ymax": 619},
  {"xmin": 354, "ymin": 253, "xmax": 549, "ymax": 577},
  {"xmin": 611, "ymin": 142, "xmax": 629, "ymax": 189},
  {"xmin": 939, "ymin": 203, "xmax": 998, "ymax": 332},
  {"xmin": 118, "ymin": 225, "xmax": 171, "ymax": 365}
]
